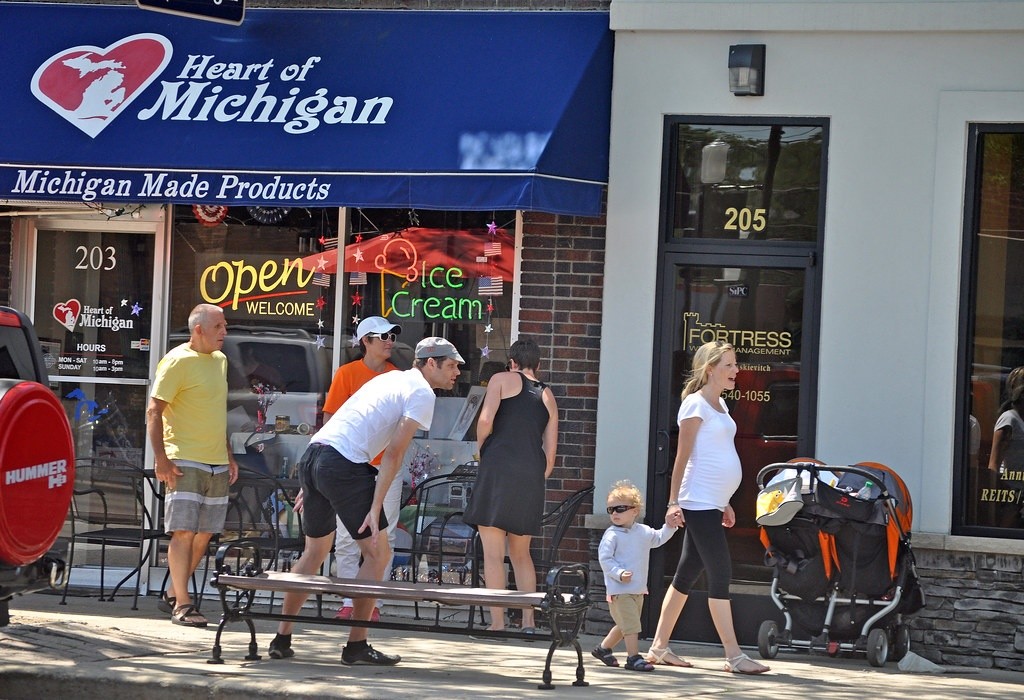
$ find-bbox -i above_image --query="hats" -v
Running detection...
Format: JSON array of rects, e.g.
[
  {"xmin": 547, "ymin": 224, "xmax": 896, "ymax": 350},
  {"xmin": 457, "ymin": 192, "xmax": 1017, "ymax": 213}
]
[
  {"xmin": 415, "ymin": 337, "xmax": 466, "ymax": 364},
  {"xmin": 356, "ymin": 316, "xmax": 401, "ymax": 342}
]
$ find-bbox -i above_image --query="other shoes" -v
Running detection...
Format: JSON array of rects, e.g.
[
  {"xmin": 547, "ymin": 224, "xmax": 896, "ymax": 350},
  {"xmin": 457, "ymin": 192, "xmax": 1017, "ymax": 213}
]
[
  {"xmin": 522, "ymin": 627, "xmax": 535, "ymax": 642},
  {"xmin": 469, "ymin": 626, "xmax": 507, "ymax": 640},
  {"xmin": 332, "ymin": 607, "xmax": 353, "ymax": 619},
  {"xmin": 370, "ymin": 607, "xmax": 380, "ymax": 621}
]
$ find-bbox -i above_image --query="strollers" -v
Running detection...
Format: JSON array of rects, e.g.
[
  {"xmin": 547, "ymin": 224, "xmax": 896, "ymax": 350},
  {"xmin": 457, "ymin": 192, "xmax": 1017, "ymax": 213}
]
[{"xmin": 757, "ymin": 457, "xmax": 927, "ymax": 667}]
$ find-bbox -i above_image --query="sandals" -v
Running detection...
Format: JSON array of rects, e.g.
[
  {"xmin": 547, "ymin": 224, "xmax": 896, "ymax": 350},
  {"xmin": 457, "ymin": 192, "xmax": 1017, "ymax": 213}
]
[
  {"xmin": 158, "ymin": 591, "xmax": 176, "ymax": 615},
  {"xmin": 648, "ymin": 646, "xmax": 685, "ymax": 667},
  {"xmin": 625, "ymin": 654, "xmax": 654, "ymax": 671},
  {"xmin": 172, "ymin": 604, "xmax": 207, "ymax": 627},
  {"xmin": 724, "ymin": 653, "xmax": 771, "ymax": 673},
  {"xmin": 591, "ymin": 643, "xmax": 619, "ymax": 667}
]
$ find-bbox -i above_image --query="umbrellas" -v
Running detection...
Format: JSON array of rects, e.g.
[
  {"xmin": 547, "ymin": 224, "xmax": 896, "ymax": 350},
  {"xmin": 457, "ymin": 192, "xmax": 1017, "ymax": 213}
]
[{"xmin": 286, "ymin": 227, "xmax": 516, "ymax": 282}]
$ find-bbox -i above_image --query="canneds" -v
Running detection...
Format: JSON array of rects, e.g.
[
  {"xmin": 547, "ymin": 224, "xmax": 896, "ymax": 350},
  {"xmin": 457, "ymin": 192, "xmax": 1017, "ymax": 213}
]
[{"xmin": 274, "ymin": 415, "xmax": 291, "ymax": 432}]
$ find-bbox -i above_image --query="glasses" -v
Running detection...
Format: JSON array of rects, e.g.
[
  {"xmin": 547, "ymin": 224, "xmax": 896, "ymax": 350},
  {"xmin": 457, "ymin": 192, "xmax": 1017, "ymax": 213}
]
[
  {"xmin": 505, "ymin": 360, "xmax": 516, "ymax": 371},
  {"xmin": 369, "ymin": 332, "xmax": 396, "ymax": 342},
  {"xmin": 607, "ymin": 506, "xmax": 635, "ymax": 514}
]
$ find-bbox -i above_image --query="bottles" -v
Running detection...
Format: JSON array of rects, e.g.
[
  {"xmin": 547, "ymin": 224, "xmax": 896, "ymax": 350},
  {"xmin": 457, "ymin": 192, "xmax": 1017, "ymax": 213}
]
[
  {"xmin": 274, "ymin": 415, "xmax": 290, "ymax": 432},
  {"xmin": 855, "ymin": 481, "xmax": 873, "ymax": 500}
]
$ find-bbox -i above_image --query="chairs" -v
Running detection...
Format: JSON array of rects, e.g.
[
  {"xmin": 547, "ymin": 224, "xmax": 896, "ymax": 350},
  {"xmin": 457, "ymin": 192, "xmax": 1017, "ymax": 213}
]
[{"xmin": 60, "ymin": 456, "xmax": 596, "ymax": 627}]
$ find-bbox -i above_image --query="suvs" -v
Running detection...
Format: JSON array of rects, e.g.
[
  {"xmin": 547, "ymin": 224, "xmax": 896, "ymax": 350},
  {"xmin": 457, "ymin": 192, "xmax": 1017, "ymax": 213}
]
[
  {"xmin": 167, "ymin": 324, "xmax": 415, "ymax": 436},
  {"xmin": 673, "ymin": 354, "xmax": 803, "ymax": 564},
  {"xmin": 0, "ymin": 306, "xmax": 77, "ymax": 627}
]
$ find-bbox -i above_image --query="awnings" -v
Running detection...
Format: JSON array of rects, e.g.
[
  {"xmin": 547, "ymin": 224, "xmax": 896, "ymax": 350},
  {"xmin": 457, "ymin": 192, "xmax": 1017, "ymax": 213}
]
[{"xmin": 0, "ymin": 0, "xmax": 614, "ymax": 218}]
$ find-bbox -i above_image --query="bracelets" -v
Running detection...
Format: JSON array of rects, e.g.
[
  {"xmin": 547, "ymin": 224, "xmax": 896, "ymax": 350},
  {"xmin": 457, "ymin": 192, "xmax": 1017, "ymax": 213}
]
[{"xmin": 667, "ymin": 501, "xmax": 679, "ymax": 508}]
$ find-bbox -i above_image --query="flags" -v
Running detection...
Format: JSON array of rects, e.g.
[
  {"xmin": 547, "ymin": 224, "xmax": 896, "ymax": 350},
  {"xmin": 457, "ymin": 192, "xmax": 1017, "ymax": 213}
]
[
  {"xmin": 312, "ymin": 273, "xmax": 330, "ymax": 287},
  {"xmin": 484, "ymin": 243, "xmax": 502, "ymax": 257},
  {"xmin": 477, "ymin": 276, "xmax": 503, "ymax": 295},
  {"xmin": 349, "ymin": 272, "xmax": 367, "ymax": 285}
]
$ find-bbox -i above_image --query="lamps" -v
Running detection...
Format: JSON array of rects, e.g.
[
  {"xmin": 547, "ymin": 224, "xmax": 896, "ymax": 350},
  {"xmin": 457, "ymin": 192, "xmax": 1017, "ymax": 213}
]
[{"xmin": 728, "ymin": 44, "xmax": 766, "ymax": 97}]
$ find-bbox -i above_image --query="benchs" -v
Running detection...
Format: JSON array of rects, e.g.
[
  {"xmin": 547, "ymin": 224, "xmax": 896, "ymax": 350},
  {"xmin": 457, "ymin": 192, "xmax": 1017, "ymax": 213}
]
[{"xmin": 210, "ymin": 539, "xmax": 591, "ymax": 690}]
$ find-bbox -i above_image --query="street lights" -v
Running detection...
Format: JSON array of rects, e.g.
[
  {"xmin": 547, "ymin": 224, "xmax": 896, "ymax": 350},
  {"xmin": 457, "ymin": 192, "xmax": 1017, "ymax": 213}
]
[{"xmin": 703, "ymin": 125, "xmax": 785, "ymax": 359}]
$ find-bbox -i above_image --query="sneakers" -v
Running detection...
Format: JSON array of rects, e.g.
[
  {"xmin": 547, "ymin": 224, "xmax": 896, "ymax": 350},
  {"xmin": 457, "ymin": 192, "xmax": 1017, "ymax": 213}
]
[
  {"xmin": 342, "ymin": 644, "xmax": 401, "ymax": 666},
  {"xmin": 269, "ymin": 643, "xmax": 294, "ymax": 659}
]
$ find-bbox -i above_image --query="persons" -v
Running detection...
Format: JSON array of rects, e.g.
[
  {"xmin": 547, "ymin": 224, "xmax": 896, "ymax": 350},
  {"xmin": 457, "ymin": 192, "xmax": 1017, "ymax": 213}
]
[
  {"xmin": 323, "ymin": 316, "xmax": 404, "ymax": 622},
  {"xmin": 148, "ymin": 304, "xmax": 237, "ymax": 626},
  {"xmin": 463, "ymin": 340, "xmax": 558, "ymax": 643},
  {"xmin": 645, "ymin": 341, "xmax": 771, "ymax": 673},
  {"xmin": 270, "ymin": 333, "xmax": 465, "ymax": 665},
  {"xmin": 592, "ymin": 479, "xmax": 682, "ymax": 672},
  {"xmin": 987, "ymin": 367, "xmax": 1024, "ymax": 498}
]
